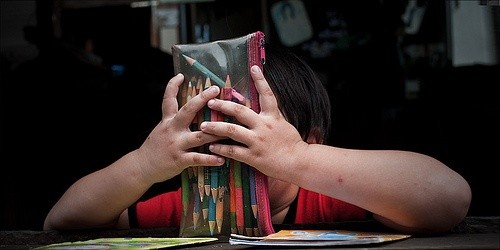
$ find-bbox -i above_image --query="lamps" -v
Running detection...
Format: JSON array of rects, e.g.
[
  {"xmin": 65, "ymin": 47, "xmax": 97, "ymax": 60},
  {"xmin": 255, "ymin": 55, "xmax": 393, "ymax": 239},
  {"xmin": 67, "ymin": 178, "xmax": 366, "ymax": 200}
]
[{"xmin": 450, "ymin": 0, "xmax": 499, "ymax": 66}]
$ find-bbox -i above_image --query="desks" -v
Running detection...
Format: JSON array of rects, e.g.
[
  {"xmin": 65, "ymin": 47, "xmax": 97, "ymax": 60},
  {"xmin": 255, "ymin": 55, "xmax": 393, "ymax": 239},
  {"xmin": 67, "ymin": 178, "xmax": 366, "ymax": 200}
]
[{"xmin": 0, "ymin": 216, "xmax": 500, "ymax": 250}]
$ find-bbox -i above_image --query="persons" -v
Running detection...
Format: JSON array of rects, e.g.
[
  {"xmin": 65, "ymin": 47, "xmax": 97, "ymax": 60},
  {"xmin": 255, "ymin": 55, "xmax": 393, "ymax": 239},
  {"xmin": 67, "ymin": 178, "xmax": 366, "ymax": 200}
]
[{"xmin": 43, "ymin": 47, "xmax": 472, "ymax": 235}]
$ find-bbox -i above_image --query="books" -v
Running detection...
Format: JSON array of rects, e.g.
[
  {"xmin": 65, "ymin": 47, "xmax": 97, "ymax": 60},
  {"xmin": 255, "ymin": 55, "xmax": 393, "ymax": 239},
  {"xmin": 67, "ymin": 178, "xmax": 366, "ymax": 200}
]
[
  {"xmin": 229, "ymin": 229, "xmax": 411, "ymax": 246},
  {"xmin": 35, "ymin": 237, "xmax": 218, "ymax": 250}
]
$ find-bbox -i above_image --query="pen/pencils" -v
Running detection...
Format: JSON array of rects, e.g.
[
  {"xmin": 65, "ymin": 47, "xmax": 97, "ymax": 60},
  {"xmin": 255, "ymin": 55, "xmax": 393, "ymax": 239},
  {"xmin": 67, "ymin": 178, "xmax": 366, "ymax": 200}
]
[{"xmin": 172, "ymin": 30, "xmax": 276, "ymax": 239}]
